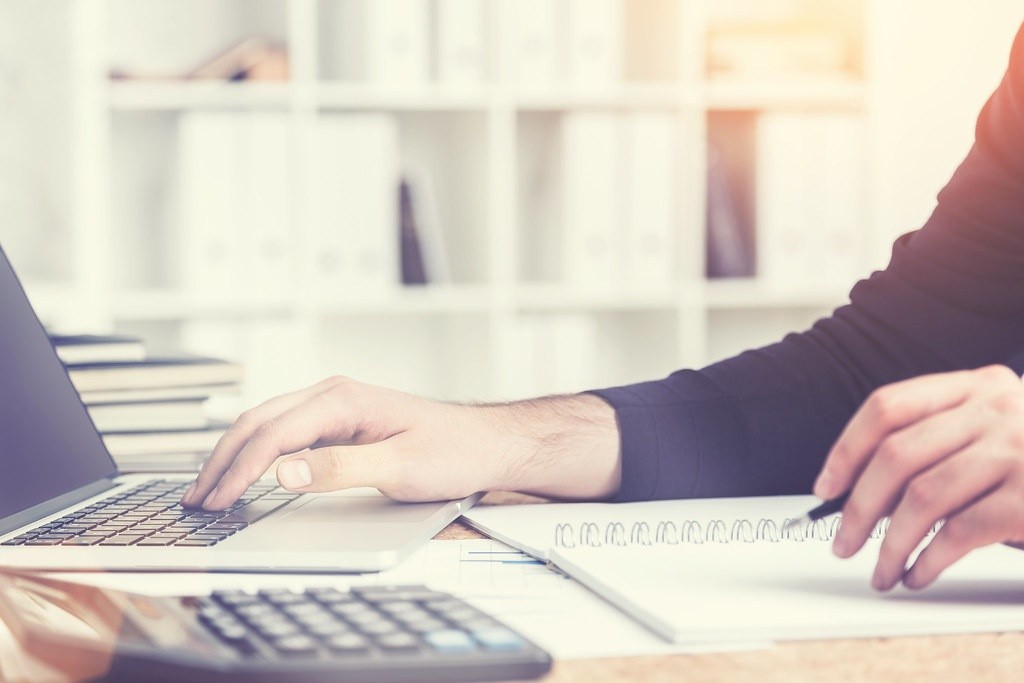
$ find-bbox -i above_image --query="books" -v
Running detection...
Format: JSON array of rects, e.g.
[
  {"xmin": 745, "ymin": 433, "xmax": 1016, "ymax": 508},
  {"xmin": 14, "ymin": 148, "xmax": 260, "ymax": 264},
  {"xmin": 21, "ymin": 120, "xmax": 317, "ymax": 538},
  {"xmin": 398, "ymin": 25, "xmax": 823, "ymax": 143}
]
[
  {"xmin": 452, "ymin": 487, "xmax": 1024, "ymax": 653},
  {"xmin": 34, "ymin": 327, "xmax": 262, "ymax": 482}
]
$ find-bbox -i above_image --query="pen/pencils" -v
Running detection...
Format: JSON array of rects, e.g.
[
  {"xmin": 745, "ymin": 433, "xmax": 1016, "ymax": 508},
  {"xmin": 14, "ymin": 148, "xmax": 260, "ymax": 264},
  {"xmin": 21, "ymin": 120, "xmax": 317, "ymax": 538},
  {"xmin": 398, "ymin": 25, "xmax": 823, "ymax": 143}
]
[{"xmin": 784, "ymin": 489, "xmax": 850, "ymax": 530}]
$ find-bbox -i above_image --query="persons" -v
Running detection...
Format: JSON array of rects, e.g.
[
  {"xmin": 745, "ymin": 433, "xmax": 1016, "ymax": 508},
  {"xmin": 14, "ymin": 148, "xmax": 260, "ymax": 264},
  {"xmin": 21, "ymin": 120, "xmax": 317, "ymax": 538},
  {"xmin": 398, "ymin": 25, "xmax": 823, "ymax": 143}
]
[{"xmin": 181, "ymin": 16, "xmax": 1022, "ymax": 595}]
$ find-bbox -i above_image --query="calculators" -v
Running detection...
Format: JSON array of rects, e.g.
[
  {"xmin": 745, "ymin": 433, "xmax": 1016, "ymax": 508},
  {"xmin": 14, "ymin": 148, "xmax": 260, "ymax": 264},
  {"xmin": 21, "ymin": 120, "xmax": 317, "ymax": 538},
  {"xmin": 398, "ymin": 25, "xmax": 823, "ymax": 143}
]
[{"xmin": 2, "ymin": 567, "xmax": 556, "ymax": 683}]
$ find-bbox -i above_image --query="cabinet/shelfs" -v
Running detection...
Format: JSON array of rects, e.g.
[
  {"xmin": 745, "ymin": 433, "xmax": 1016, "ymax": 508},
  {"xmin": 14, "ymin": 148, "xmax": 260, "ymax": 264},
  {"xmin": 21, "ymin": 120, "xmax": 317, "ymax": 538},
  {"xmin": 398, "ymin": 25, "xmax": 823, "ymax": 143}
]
[{"xmin": 77, "ymin": 3, "xmax": 878, "ymax": 312}]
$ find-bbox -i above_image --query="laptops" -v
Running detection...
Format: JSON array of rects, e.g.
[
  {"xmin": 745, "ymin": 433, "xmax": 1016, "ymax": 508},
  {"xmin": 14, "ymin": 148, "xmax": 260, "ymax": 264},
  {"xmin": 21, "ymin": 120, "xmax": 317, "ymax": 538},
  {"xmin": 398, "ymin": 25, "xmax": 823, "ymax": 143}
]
[{"xmin": 0, "ymin": 248, "xmax": 489, "ymax": 574}]
